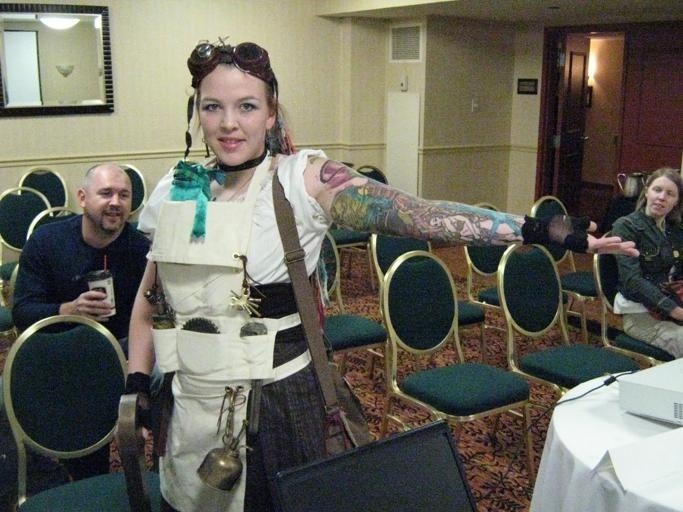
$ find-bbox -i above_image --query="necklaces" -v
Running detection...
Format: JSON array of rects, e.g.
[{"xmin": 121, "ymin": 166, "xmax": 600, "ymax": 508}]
[
  {"xmin": 216, "ymin": 148, "xmax": 268, "ymax": 172},
  {"xmin": 222, "ymin": 173, "xmax": 252, "ymax": 202}
]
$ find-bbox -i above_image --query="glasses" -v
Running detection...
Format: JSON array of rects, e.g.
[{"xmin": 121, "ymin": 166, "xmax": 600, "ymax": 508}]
[{"xmin": 187, "ymin": 42, "xmax": 274, "ymax": 88}]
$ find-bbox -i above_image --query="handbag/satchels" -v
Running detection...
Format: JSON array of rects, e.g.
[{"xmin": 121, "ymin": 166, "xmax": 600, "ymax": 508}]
[
  {"xmin": 649, "ymin": 281, "xmax": 682, "ymax": 327},
  {"xmin": 324, "ymin": 357, "xmax": 369, "ymax": 458}
]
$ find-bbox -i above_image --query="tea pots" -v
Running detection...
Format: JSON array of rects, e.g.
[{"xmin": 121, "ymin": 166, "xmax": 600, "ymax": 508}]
[{"xmin": 616, "ymin": 171, "xmax": 647, "ymax": 206}]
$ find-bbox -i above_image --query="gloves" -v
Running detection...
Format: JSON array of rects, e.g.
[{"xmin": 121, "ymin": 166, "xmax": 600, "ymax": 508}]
[
  {"xmin": 110, "ymin": 372, "xmax": 154, "ymax": 472},
  {"xmin": 521, "ymin": 214, "xmax": 591, "ymax": 255}
]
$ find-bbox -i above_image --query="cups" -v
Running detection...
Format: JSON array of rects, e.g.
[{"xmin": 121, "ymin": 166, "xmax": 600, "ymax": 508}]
[{"xmin": 86, "ymin": 270, "xmax": 118, "ymax": 319}]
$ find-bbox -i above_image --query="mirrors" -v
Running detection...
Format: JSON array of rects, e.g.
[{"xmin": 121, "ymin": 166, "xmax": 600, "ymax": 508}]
[{"xmin": 0, "ymin": 2, "xmax": 115, "ymax": 120}]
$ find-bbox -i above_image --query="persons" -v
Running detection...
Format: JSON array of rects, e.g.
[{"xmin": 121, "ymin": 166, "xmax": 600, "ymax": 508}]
[
  {"xmin": 12, "ymin": 163, "xmax": 167, "ymax": 484},
  {"xmin": 610, "ymin": 168, "xmax": 683, "ymax": 362},
  {"xmin": 107, "ymin": 36, "xmax": 641, "ymax": 512}
]
